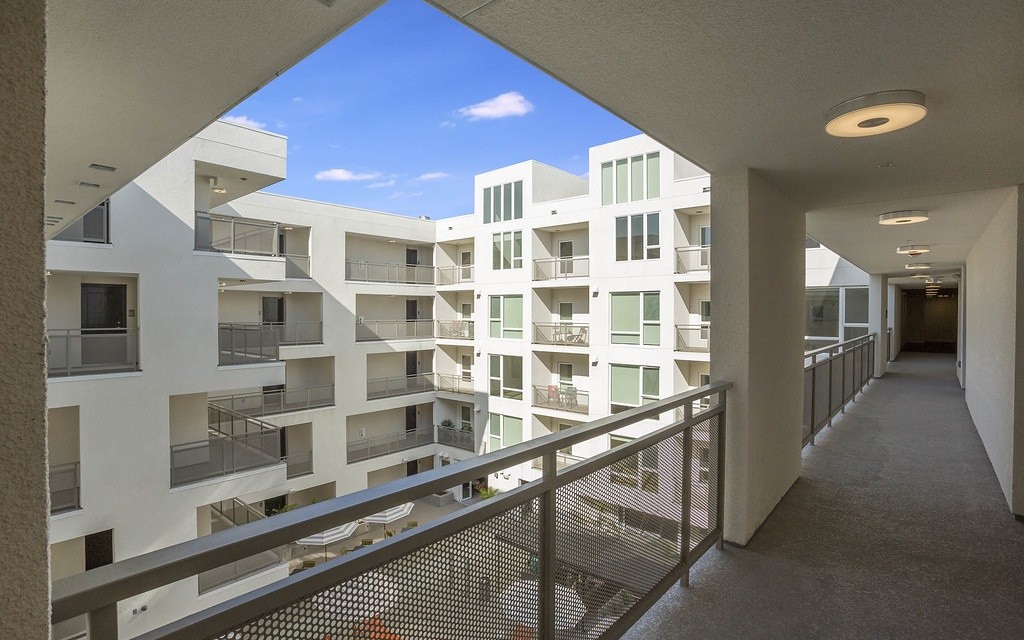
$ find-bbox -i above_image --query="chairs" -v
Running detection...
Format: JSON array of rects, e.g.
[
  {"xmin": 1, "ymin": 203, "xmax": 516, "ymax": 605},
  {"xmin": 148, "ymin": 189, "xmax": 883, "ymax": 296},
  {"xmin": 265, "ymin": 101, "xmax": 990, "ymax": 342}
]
[
  {"xmin": 302, "ymin": 521, "xmax": 418, "ymax": 569},
  {"xmin": 547, "ymin": 385, "xmax": 578, "ymax": 409},
  {"xmin": 552, "ymin": 321, "xmax": 587, "ymax": 344},
  {"xmin": 450, "ymin": 320, "xmax": 464, "ymax": 339}
]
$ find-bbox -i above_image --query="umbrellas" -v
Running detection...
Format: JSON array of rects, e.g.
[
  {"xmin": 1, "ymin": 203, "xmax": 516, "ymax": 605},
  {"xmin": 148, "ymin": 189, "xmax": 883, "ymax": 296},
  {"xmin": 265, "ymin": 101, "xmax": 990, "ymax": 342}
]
[
  {"xmin": 294, "ymin": 520, "xmax": 359, "ymax": 562},
  {"xmin": 359, "ymin": 502, "xmax": 415, "ymax": 540},
  {"xmin": 500, "ymin": 579, "xmax": 586, "ymax": 630}
]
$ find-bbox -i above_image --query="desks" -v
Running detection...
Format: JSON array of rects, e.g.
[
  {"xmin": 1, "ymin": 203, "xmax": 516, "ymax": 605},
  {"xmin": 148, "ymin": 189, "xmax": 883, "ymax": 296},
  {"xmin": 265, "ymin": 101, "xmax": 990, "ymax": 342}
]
[
  {"xmin": 401, "ymin": 527, "xmax": 414, "ymax": 534},
  {"xmin": 289, "ymin": 569, "xmax": 308, "ymax": 577},
  {"xmin": 352, "ymin": 546, "xmax": 367, "ymax": 552}
]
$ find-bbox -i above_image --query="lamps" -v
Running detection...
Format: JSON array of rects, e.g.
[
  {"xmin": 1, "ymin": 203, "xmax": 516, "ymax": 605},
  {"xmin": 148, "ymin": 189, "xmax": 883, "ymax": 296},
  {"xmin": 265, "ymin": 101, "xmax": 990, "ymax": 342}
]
[
  {"xmin": 904, "ymin": 263, "xmax": 949, "ymax": 298},
  {"xmin": 895, "ymin": 245, "xmax": 931, "ymax": 259},
  {"xmin": 878, "ymin": 210, "xmax": 929, "ymax": 225},
  {"xmin": 823, "ymin": 90, "xmax": 928, "ymax": 138}
]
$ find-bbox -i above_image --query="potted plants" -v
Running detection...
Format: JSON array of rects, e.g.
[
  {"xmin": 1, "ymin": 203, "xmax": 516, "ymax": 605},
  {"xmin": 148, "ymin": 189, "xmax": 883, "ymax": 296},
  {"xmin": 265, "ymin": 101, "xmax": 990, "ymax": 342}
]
[{"xmin": 441, "ymin": 419, "xmax": 473, "ymax": 447}]
